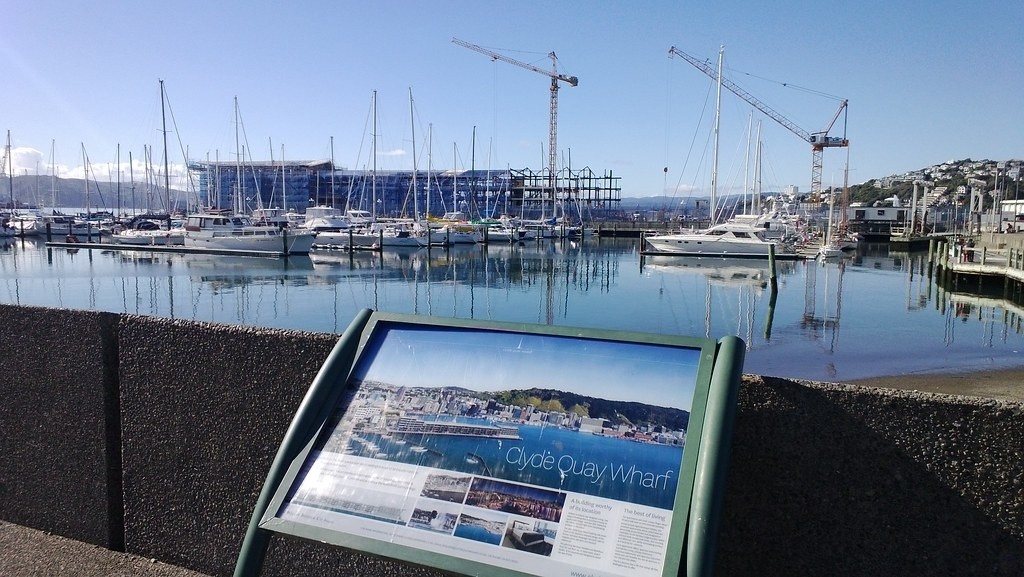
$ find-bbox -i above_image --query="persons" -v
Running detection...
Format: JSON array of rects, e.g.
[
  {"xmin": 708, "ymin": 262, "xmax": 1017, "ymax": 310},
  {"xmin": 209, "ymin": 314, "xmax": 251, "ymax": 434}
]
[
  {"xmin": 963, "ymin": 239, "xmax": 975, "ymax": 262},
  {"xmin": 1007, "ymin": 223, "xmax": 1012, "ymax": 231},
  {"xmin": 781, "ymin": 233, "xmax": 786, "ymax": 241},
  {"xmin": 959, "ymin": 234, "xmax": 964, "ymax": 253}
]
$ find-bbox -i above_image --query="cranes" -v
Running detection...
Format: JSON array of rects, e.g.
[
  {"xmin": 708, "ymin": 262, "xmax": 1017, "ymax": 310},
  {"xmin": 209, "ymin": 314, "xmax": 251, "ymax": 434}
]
[
  {"xmin": 450, "ymin": 35, "xmax": 578, "ymax": 206},
  {"xmin": 668, "ymin": 44, "xmax": 850, "ymax": 215}
]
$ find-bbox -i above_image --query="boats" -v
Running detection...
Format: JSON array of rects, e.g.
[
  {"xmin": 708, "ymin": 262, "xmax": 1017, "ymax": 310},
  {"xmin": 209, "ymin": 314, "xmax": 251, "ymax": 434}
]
[{"xmin": 332, "ymin": 420, "xmax": 481, "ymax": 465}]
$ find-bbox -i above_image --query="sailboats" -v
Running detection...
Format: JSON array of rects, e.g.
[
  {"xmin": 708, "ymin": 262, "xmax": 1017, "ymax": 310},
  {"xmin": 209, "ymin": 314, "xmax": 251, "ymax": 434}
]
[
  {"xmin": 643, "ymin": 40, "xmax": 865, "ymax": 262},
  {"xmin": 0, "ymin": 78, "xmax": 600, "ymax": 258}
]
